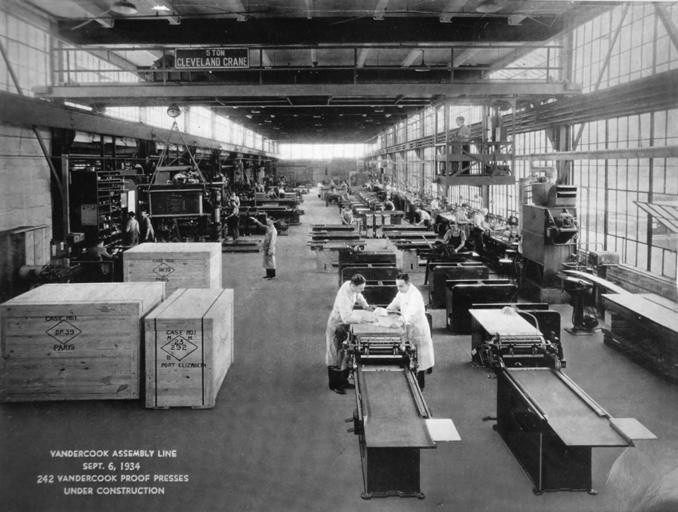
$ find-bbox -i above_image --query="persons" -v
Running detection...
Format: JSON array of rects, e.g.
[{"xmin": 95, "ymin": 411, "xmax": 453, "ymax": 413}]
[
  {"xmin": 317, "ymin": 181, "xmax": 325, "ymax": 198},
  {"xmin": 141, "ymin": 210, "xmax": 155, "ymax": 242},
  {"xmin": 456, "ymin": 117, "xmax": 471, "ymax": 174},
  {"xmin": 267, "ymin": 187, "xmax": 277, "ymax": 199},
  {"xmin": 125, "ymin": 211, "xmax": 140, "ymax": 245},
  {"xmin": 249, "ymin": 216, "xmax": 278, "ymax": 280},
  {"xmin": 249, "ymin": 182, "xmax": 264, "ymax": 193},
  {"xmin": 278, "ymin": 184, "xmax": 285, "ymax": 194},
  {"xmin": 386, "ymin": 274, "xmax": 434, "ymax": 392},
  {"xmin": 325, "ymin": 274, "xmax": 376, "ymax": 395},
  {"xmin": 225, "ymin": 200, "xmax": 240, "ymax": 240},
  {"xmin": 87, "ymin": 240, "xmax": 119, "ymax": 273},
  {"xmin": 328, "ymin": 178, "xmax": 336, "ymax": 187},
  {"xmin": 227, "ymin": 192, "xmax": 240, "ymax": 208},
  {"xmin": 384, "ymin": 199, "xmax": 395, "ymax": 212},
  {"xmin": 431, "ymin": 195, "xmax": 442, "ymax": 222},
  {"xmin": 413, "ymin": 207, "xmax": 435, "ymax": 231},
  {"xmin": 474, "ymin": 208, "xmax": 488, "ymax": 256},
  {"xmin": 443, "ymin": 221, "xmax": 466, "ymax": 254},
  {"xmin": 340, "ymin": 180, "xmax": 349, "ymax": 193},
  {"xmin": 457, "ymin": 203, "xmax": 473, "ymax": 234}
]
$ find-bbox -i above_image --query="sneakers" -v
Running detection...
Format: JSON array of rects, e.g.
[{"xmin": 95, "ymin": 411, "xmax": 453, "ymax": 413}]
[
  {"xmin": 262, "ymin": 276, "xmax": 268, "ymax": 278},
  {"xmin": 332, "ymin": 388, "xmax": 346, "ymax": 394},
  {"xmin": 347, "ymin": 383, "xmax": 355, "ymax": 389},
  {"xmin": 268, "ymin": 276, "xmax": 272, "ymax": 280}
]
